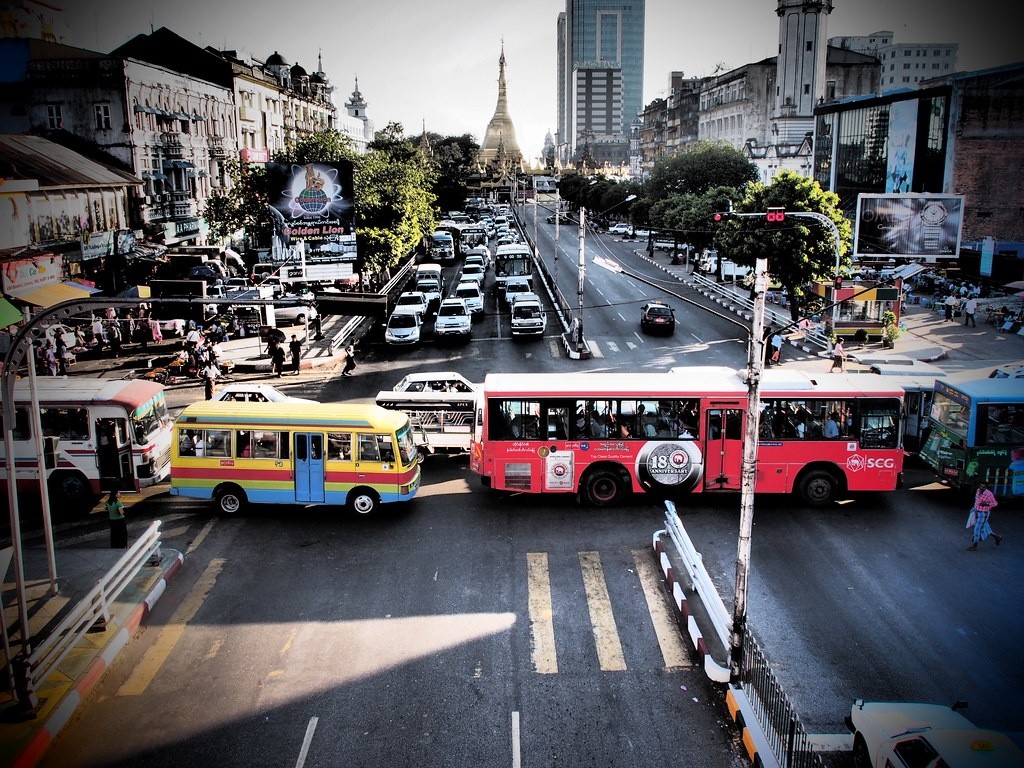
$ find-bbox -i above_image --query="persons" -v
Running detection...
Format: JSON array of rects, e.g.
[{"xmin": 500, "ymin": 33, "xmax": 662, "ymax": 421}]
[
  {"xmin": 363, "ymin": 265, "xmax": 390, "ymax": 293},
  {"xmin": 342, "ymin": 338, "xmax": 356, "ymax": 374},
  {"xmin": 15, "ymin": 410, "xmax": 155, "ymax": 474},
  {"xmin": 912, "ymin": 270, "xmax": 981, "ymax": 327},
  {"xmin": 829, "ymin": 338, "xmax": 846, "ymax": 373},
  {"xmin": 264, "ymin": 334, "xmax": 302, "ymax": 378},
  {"xmin": 216, "ymin": 276, "xmax": 223, "ymax": 285},
  {"xmin": 37, "ymin": 303, "xmax": 162, "ymax": 376},
  {"xmin": 174, "ymin": 312, "xmax": 245, "ymax": 400},
  {"xmin": 761, "ymin": 326, "xmax": 783, "ymax": 365},
  {"xmin": 966, "ymin": 480, "xmax": 1003, "ymax": 551},
  {"xmin": 180, "ymin": 429, "xmax": 351, "ymax": 459},
  {"xmin": 586, "ymin": 404, "xmax": 854, "ymax": 441},
  {"xmin": 451, "ymin": 381, "xmax": 458, "ymax": 392},
  {"xmin": 423, "ymin": 382, "xmax": 446, "ymax": 392}
]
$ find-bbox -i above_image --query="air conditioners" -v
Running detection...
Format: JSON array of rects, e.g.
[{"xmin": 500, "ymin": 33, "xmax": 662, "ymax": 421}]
[
  {"xmin": 165, "ymin": 194, "xmax": 171, "ymax": 201},
  {"xmin": 144, "ymin": 196, "xmax": 152, "ymax": 205},
  {"xmin": 154, "ymin": 195, "xmax": 161, "ymax": 202}
]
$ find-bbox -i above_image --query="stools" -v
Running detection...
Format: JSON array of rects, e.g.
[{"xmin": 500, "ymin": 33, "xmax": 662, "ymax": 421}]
[{"xmin": 910, "ymin": 296, "xmax": 960, "ymax": 316}]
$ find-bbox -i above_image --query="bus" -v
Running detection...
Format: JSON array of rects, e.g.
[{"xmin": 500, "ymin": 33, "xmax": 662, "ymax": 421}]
[
  {"xmin": 491, "ymin": 243, "xmax": 533, "ymax": 292},
  {"xmin": 0, "ymin": 376, "xmax": 173, "ymax": 517},
  {"xmin": 471, "ymin": 368, "xmax": 912, "ymax": 513},
  {"xmin": 917, "ymin": 378, "xmax": 1024, "ymax": 499},
  {"xmin": 168, "ymin": 400, "xmax": 422, "ymax": 527}
]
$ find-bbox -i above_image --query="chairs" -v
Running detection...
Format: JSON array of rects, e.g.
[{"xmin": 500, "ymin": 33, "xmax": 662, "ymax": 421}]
[{"xmin": 558, "ymin": 415, "xmax": 825, "ymax": 437}]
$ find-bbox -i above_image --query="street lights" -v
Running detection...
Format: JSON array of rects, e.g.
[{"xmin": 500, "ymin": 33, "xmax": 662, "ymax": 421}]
[{"xmin": 575, "ymin": 192, "xmax": 637, "ymax": 350}]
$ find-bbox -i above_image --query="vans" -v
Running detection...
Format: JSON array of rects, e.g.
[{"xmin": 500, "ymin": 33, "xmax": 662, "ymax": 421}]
[{"xmin": 841, "ymin": 355, "xmax": 948, "ymax": 452}]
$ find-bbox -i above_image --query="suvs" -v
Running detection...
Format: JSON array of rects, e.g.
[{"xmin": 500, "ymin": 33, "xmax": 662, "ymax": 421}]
[
  {"xmin": 432, "ymin": 297, "xmax": 474, "ymax": 342},
  {"xmin": 545, "ymin": 212, "xmax": 573, "ymax": 225}
]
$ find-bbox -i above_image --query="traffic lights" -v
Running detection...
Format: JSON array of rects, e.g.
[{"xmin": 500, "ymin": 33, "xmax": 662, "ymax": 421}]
[
  {"xmin": 712, "ymin": 211, "xmax": 739, "ymax": 222},
  {"xmin": 765, "ymin": 207, "xmax": 785, "ymax": 221},
  {"xmin": 835, "ymin": 275, "xmax": 843, "ymax": 290}
]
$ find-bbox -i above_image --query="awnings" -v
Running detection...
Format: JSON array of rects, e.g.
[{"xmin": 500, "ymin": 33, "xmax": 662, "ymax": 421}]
[
  {"xmin": 124, "ymin": 239, "xmax": 167, "ymax": 263},
  {"xmin": 0, "ymin": 298, "xmax": 24, "ymax": 328},
  {"xmin": 8, "ymin": 279, "xmax": 103, "ymax": 308}
]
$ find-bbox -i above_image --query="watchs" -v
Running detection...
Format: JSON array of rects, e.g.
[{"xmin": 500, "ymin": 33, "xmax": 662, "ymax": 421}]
[{"xmin": 920, "ymin": 200, "xmax": 948, "ymax": 250}]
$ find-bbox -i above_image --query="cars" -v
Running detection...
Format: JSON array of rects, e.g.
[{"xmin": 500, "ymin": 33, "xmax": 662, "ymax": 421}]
[
  {"xmin": 382, "ymin": 309, "xmax": 424, "ymax": 348},
  {"xmin": 199, "ymin": 382, "xmax": 321, "ymax": 405},
  {"xmin": 988, "ymin": 364, "xmax": 1024, "ymax": 380},
  {"xmin": 606, "ymin": 222, "xmax": 788, "ymax": 290},
  {"xmin": 393, "ymin": 371, "xmax": 484, "ymax": 394},
  {"xmin": 275, "ymin": 300, "xmax": 318, "ymax": 326},
  {"xmin": 153, "ymin": 318, "xmax": 188, "ymax": 337},
  {"xmin": 639, "ymin": 299, "xmax": 676, "ymax": 338},
  {"xmin": 28, "ymin": 323, "xmax": 77, "ymax": 352},
  {"xmin": 392, "ymin": 196, "xmax": 547, "ymax": 337}
]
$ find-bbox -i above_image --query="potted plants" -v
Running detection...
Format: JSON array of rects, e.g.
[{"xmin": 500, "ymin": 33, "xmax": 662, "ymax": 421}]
[
  {"xmin": 881, "ymin": 311, "xmax": 900, "ymax": 348},
  {"xmin": 855, "ymin": 329, "xmax": 869, "ymax": 348}
]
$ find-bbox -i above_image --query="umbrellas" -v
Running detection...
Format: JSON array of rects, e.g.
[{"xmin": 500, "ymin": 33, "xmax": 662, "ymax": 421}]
[{"xmin": 1004, "ymin": 281, "xmax": 1024, "ymax": 289}]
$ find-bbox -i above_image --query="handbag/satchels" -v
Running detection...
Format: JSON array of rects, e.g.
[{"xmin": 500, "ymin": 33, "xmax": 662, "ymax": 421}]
[
  {"xmin": 966, "ymin": 511, "xmax": 976, "ymax": 529},
  {"xmin": 772, "ymin": 350, "xmax": 781, "ymax": 361}
]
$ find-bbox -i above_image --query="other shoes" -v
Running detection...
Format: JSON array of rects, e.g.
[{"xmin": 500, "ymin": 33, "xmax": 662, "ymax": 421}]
[
  {"xmin": 777, "ymin": 362, "xmax": 782, "ymax": 366},
  {"xmin": 944, "ymin": 319, "xmax": 949, "ymax": 322},
  {"xmin": 967, "ymin": 544, "xmax": 977, "ymax": 551},
  {"xmin": 996, "ymin": 536, "xmax": 1003, "ymax": 546},
  {"xmin": 951, "ymin": 317, "xmax": 953, "ymax": 321},
  {"xmin": 972, "ymin": 323, "xmax": 976, "ymax": 328},
  {"xmin": 963, "ymin": 323, "xmax": 968, "ymax": 326}
]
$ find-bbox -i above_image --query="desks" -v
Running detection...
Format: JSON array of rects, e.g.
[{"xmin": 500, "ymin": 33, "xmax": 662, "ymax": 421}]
[{"xmin": 985, "ymin": 309, "xmax": 1000, "ymax": 324}]
